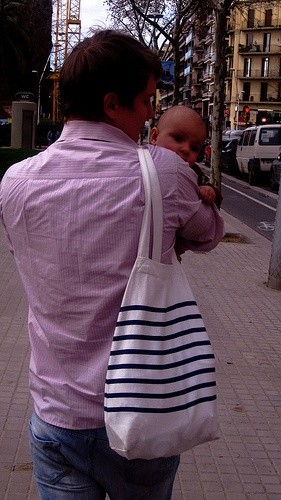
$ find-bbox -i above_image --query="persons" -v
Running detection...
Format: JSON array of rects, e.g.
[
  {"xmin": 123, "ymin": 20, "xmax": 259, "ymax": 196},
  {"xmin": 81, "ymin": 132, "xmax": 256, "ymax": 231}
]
[
  {"xmin": 150, "ymin": 101, "xmax": 223, "ymax": 212},
  {"xmin": 0, "ymin": 28, "xmax": 224, "ymax": 500}
]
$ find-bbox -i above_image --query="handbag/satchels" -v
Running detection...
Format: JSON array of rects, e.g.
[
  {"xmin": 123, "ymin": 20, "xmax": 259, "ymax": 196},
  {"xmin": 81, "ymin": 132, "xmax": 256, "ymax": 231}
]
[{"xmin": 104, "ymin": 146, "xmax": 223, "ymax": 461}]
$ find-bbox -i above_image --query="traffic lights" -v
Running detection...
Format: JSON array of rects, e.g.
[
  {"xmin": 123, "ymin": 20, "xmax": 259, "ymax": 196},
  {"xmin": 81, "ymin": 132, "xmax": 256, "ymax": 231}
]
[{"xmin": 243, "ymin": 105, "xmax": 250, "ymax": 112}]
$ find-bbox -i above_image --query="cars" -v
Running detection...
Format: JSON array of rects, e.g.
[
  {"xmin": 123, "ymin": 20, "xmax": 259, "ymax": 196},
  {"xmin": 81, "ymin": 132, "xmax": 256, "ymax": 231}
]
[{"xmin": 203, "ymin": 126, "xmax": 239, "ymax": 173}]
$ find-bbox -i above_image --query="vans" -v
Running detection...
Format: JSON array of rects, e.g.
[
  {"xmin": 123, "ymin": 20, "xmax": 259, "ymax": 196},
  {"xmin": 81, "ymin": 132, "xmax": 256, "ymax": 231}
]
[{"xmin": 236, "ymin": 124, "xmax": 281, "ymax": 185}]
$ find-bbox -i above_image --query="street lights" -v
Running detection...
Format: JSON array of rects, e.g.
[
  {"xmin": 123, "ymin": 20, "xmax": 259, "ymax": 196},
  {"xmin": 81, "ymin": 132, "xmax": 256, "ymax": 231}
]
[{"xmin": 236, "ymin": 90, "xmax": 245, "ymax": 131}]
